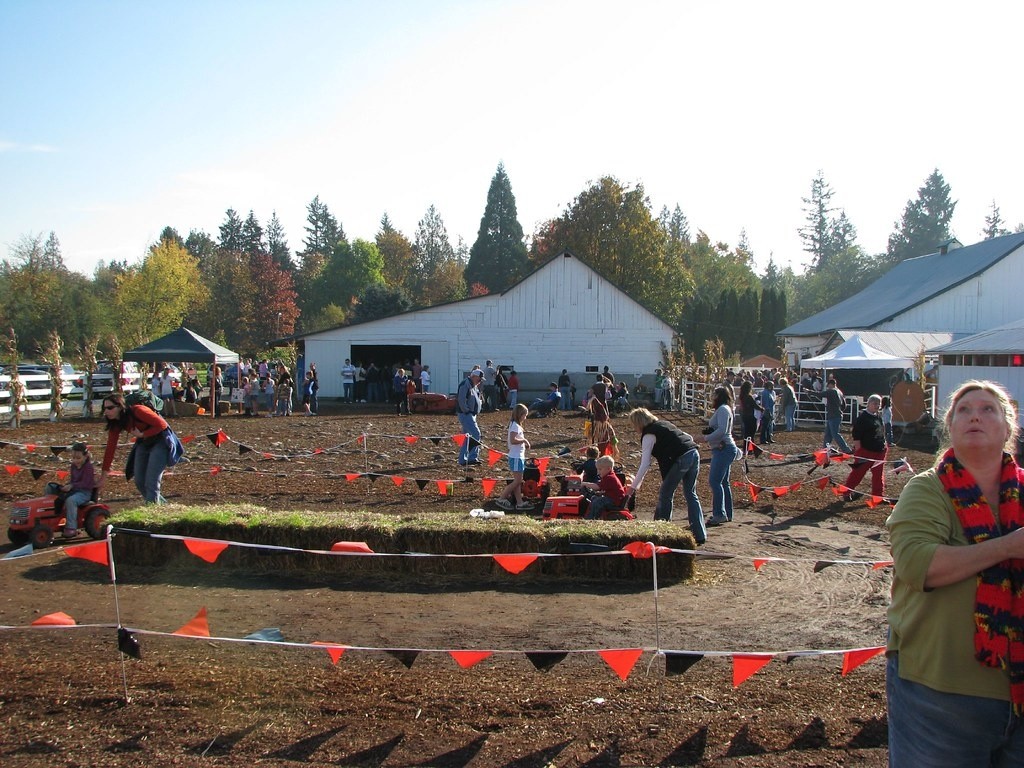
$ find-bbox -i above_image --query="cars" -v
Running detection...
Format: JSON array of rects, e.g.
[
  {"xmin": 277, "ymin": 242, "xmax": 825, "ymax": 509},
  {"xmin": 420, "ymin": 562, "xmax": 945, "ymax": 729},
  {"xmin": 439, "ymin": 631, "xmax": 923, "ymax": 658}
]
[
  {"xmin": 164, "ymin": 363, "xmax": 182, "ymax": 383},
  {"xmin": 77, "ymin": 362, "xmax": 138, "ymax": 396},
  {"xmin": 206, "ymin": 361, "xmax": 235, "ymax": 387},
  {"xmin": 0, "ymin": 370, "xmax": 52, "ymax": 401}
]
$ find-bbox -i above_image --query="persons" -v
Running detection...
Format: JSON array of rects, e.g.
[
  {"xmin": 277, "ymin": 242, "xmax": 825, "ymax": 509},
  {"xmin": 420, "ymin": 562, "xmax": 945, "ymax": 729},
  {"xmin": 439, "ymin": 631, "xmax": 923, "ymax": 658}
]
[
  {"xmin": 496, "ymin": 404, "xmax": 536, "ymax": 509},
  {"xmin": 886, "ymin": 379, "xmax": 1024, "ymax": 768},
  {"xmin": 803, "ymin": 373, "xmax": 897, "ymax": 453},
  {"xmin": 574, "ymin": 446, "xmax": 625, "ymax": 521},
  {"xmin": 655, "ymin": 365, "xmax": 836, "ymax": 453},
  {"xmin": 703, "ymin": 386, "xmax": 737, "ymax": 525},
  {"xmin": 95, "ymin": 394, "xmax": 186, "ymax": 506},
  {"xmin": 469, "ymin": 360, "xmax": 629, "ymax": 422},
  {"xmin": 626, "ymin": 408, "xmax": 707, "ymax": 544},
  {"xmin": 457, "ymin": 370, "xmax": 483, "ymax": 466},
  {"xmin": 151, "ymin": 358, "xmax": 432, "ymax": 416},
  {"xmin": 842, "ymin": 394, "xmax": 891, "ymax": 504},
  {"xmin": 46, "ymin": 441, "xmax": 93, "ymax": 537}
]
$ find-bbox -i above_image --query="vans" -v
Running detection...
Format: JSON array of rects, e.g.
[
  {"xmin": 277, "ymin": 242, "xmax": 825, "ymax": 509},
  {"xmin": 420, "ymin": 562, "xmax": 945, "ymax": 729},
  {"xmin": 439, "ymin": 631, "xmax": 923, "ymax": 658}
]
[{"xmin": 3, "ymin": 363, "xmax": 76, "ymax": 402}]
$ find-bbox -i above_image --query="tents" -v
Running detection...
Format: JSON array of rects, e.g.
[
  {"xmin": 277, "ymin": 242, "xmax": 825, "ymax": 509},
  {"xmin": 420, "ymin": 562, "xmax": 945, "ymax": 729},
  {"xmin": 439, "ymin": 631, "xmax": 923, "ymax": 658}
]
[
  {"xmin": 797, "ymin": 335, "xmax": 916, "ymax": 428},
  {"xmin": 122, "ymin": 327, "xmax": 242, "ymax": 418}
]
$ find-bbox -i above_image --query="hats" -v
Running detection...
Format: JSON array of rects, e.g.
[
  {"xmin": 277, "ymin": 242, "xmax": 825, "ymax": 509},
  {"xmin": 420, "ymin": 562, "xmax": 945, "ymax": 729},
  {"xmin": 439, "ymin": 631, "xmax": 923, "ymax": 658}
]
[
  {"xmin": 727, "ymin": 372, "xmax": 736, "ymax": 376},
  {"xmin": 826, "ymin": 379, "xmax": 837, "ymax": 387},
  {"xmin": 486, "ymin": 360, "xmax": 493, "ymax": 364},
  {"xmin": 472, "ymin": 369, "xmax": 487, "ymax": 381}
]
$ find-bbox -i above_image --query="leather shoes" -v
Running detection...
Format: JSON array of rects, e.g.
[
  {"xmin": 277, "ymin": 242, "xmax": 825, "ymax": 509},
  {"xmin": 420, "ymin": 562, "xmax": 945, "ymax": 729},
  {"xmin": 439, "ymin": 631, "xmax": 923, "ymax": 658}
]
[
  {"xmin": 843, "ymin": 493, "xmax": 852, "ymax": 501},
  {"xmin": 62, "ymin": 529, "xmax": 76, "ymax": 538},
  {"xmin": 468, "ymin": 459, "xmax": 482, "ymax": 465},
  {"xmin": 767, "ymin": 438, "xmax": 776, "ymax": 443},
  {"xmin": 760, "ymin": 439, "xmax": 768, "ymax": 444},
  {"xmin": 878, "ymin": 498, "xmax": 891, "ymax": 504}
]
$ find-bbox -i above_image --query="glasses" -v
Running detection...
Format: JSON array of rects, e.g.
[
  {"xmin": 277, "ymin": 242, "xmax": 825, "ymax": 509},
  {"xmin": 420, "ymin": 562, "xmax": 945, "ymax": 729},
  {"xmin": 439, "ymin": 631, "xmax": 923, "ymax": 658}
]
[{"xmin": 103, "ymin": 405, "xmax": 120, "ymax": 411}]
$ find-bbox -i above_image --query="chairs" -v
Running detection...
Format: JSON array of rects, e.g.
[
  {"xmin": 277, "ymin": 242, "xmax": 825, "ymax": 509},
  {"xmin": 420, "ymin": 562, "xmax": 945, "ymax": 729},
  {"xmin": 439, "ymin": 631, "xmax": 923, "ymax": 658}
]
[{"xmin": 603, "ymin": 494, "xmax": 633, "ymax": 521}]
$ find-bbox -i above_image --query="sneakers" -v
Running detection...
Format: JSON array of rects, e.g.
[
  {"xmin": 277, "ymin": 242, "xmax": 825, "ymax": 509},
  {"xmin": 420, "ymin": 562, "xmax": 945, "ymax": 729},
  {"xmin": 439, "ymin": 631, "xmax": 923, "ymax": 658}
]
[
  {"xmin": 495, "ymin": 498, "xmax": 514, "ymax": 510},
  {"xmin": 516, "ymin": 500, "xmax": 535, "ymax": 510},
  {"xmin": 727, "ymin": 518, "xmax": 733, "ymax": 521},
  {"xmin": 304, "ymin": 411, "xmax": 313, "ymax": 416},
  {"xmin": 707, "ymin": 512, "xmax": 727, "ymax": 524}
]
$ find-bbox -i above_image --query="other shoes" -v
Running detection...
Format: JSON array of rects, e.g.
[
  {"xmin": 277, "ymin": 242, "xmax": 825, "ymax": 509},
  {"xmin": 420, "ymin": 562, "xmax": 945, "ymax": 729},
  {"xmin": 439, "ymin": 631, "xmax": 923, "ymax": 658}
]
[
  {"xmin": 461, "ymin": 462, "xmax": 467, "ymax": 466},
  {"xmin": 695, "ymin": 539, "xmax": 706, "ymax": 544}
]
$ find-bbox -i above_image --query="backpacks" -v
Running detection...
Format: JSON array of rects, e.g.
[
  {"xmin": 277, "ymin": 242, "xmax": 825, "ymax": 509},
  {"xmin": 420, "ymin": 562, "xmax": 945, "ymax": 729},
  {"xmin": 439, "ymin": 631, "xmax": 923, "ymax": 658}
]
[
  {"xmin": 124, "ymin": 389, "xmax": 163, "ymax": 414},
  {"xmin": 217, "ymin": 378, "xmax": 223, "ymax": 393}
]
